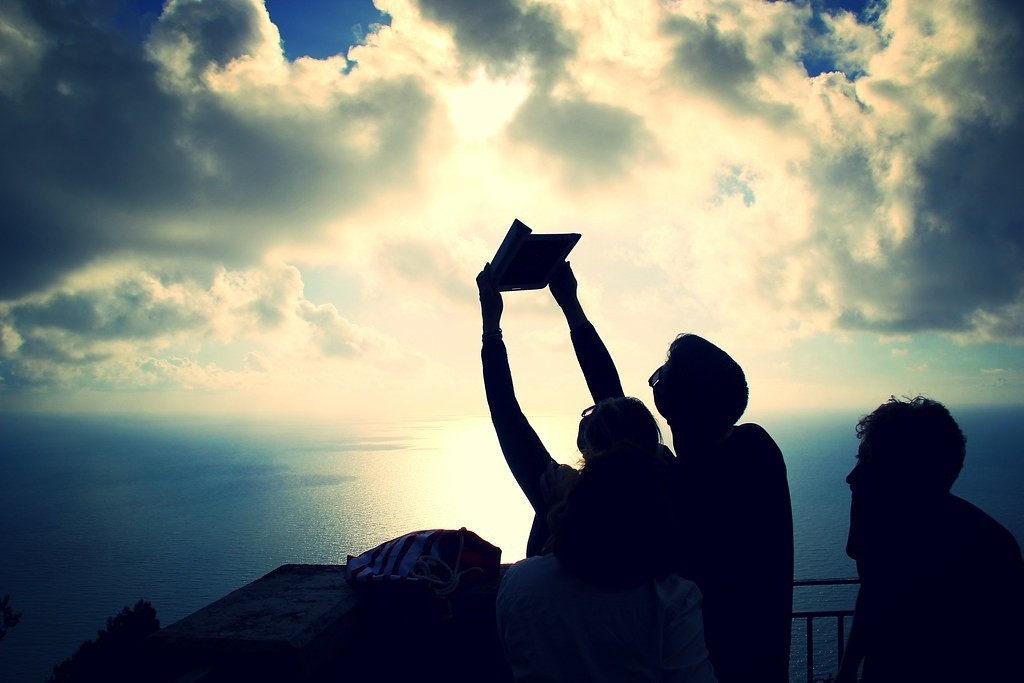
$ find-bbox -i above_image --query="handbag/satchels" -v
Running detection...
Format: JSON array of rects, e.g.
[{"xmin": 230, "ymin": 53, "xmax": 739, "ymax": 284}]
[{"xmin": 346, "ymin": 530, "xmax": 503, "ymax": 592}]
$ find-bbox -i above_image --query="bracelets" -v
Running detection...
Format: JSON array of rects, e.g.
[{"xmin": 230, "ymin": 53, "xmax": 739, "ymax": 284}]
[{"xmin": 482, "ymin": 328, "xmax": 503, "ymax": 341}]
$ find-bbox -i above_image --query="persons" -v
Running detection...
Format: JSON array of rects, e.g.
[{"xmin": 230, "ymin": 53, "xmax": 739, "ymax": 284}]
[
  {"xmin": 834, "ymin": 401, "xmax": 1023, "ymax": 683},
  {"xmin": 478, "ymin": 258, "xmax": 794, "ymax": 683}
]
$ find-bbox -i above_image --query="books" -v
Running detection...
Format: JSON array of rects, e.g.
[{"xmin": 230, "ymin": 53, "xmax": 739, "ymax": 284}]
[{"xmin": 476, "ymin": 224, "xmax": 581, "ymax": 289}]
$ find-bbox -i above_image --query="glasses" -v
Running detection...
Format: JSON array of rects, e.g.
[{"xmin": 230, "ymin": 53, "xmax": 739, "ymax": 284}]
[
  {"xmin": 649, "ymin": 367, "xmax": 683, "ymax": 410},
  {"xmin": 581, "ymin": 404, "xmax": 596, "ymax": 442}
]
